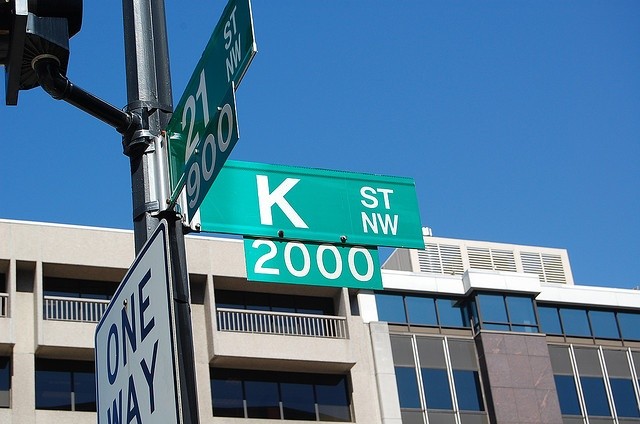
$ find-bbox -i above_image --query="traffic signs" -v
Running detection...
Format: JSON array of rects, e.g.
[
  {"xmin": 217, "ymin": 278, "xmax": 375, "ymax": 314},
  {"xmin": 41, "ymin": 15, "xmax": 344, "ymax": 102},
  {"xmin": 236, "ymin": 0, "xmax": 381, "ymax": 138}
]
[
  {"xmin": 198, "ymin": 163, "xmax": 425, "ymax": 291},
  {"xmin": 94, "ymin": 216, "xmax": 199, "ymax": 424},
  {"xmin": 167, "ymin": 0, "xmax": 258, "ymax": 224}
]
